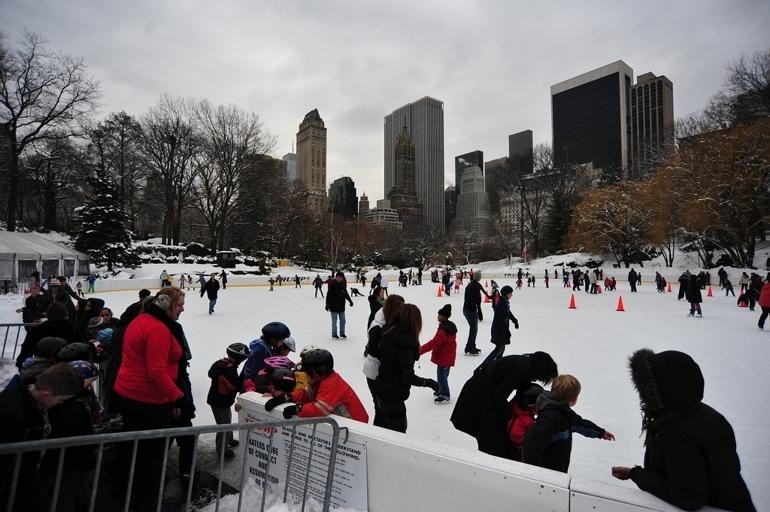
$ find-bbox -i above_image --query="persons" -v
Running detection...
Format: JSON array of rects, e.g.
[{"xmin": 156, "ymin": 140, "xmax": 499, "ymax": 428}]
[
  {"xmin": 491, "ymin": 285, "xmax": 521, "ymax": 363},
  {"xmin": 1, "ymin": 261, "xmax": 206, "ymax": 512},
  {"xmin": 462, "ymin": 270, "xmax": 491, "ymax": 356},
  {"xmin": 207, "ymin": 341, "xmax": 249, "ymax": 460},
  {"xmin": 206, "ymin": 261, "xmax": 769, "ymax": 340},
  {"xmin": 611, "ymin": 348, "xmax": 758, "ymax": 511},
  {"xmin": 522, "ymin": 374, "xmax": 615, "ymax": 474},
  {"xmin": 507, "ymin": 378, "xmax": 547, "ymax": 453},
  {"xmin": 449, "ymin": 351, "xmax": 558, "ymax": 458},
  {"xmin": 234, "ymin": 322, "xmax": 458, "ymax": 435}
]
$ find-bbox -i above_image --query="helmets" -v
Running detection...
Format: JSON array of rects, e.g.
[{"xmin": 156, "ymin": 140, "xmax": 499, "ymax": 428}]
[
  {"xmin": 302, "ymin": 349, "xmax": 333, "ymax": 368},
  {"xmin": 516, "ymin": 384, "xmax": 544, "ymax": 408},
  {"xmin": 58, "ymin": 343, "xmax": 97, "ymax": 362},
  {"xmin": 501, "ymin": 286, "xmax": 513, "ymax": 294},
  {"xmin": 69, "ymin": 360, "xmax": 99, "ymax": 389},
  {"xmin": 264, "ymin": 356, "xmax": 296, "ymax": 370},
  {"xmin": 300, "ymin": 344, "xmax": 318, "ymax": 356},
  {"xmin": 35, "ymin": 338, "xmax": 68, "ymax": 358},
  {"xmin": 97, "ymin": 328, "xmax": 114, "ymax": 348},
  {"xmin": 227, "ymin": 343, "xmax": 249, "ymax": 359},
  {"xmin": 272, "ymin": 368, "xmax": 295, "ymax": 391},
  {"xmin": 262, "ymin": 322, "xmax": 290, "ymax": 338},
  {"xmin": 283, "ymin": 338, "xmax": 295, "ymax": 352}
]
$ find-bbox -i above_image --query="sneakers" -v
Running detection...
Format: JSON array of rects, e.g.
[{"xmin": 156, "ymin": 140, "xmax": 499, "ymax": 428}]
[
  {"xmin": 434, "ymin": 391, "xmax": 441, "ymax": 395},
  {"xmin": 332, "ymin": 335, "xmax": 339, "ymax": 338},
  {"xmin": 212, "ymin": 310, "xmax": 214, "ymax": 312},
  {"xmin": 183, "ymin": 464, "xmax": 200, "ymax": 478},
  {"xmin": 435, "ymin": 396, "xmax": 450, "ymax": 402},
  {"xmin": 228, "ymin": 439, "xmax": 239, "ymax": 446},
  {"xmin": 474, "ymin": 344, "xmax": 481, "ymax": 352},
  {"xmin": 209, "ymin": 311, "xmax": 212, "ymax": 314},
  {"xmin": 340, "ymin": 334, "xmax": 346, "ymax": 338},
  {"xmin": 465, "ymin": 344, "xmax": 478, "ymax": 353},
  {"xmin": 219, "ymin": 449, "xmax": 234, "ymax": 458}
]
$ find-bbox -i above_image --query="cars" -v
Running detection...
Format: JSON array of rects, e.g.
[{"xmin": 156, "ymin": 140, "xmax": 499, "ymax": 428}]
[{"xmin": 680, "ymin": 238, "xmax": 722, "ymax": 253}]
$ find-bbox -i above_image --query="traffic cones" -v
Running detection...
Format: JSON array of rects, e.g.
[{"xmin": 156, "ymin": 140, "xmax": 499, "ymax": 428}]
[
  {"xmin": 739, "ymin": 290, "xmax": 751, "ymax": 308},
  {"xmin": 706, "ymin": 287, "xmax": 715, "ymax": 297},
  {"xmin": 568, "ymin": 293, "xmax": 578, "ymax": 309},
  {"xmin": 615, "ymin": 295, "xmax": 627, "ymax": 311},
  {"xmin": 436, "ymin": 279, "xmax": 491, "ymax": 304},
  {"xmin": 668, "ymin": 283, "xmax": 672, "ymax": 293}
]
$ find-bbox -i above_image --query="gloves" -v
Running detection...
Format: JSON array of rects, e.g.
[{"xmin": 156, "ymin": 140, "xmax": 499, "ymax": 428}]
[
  {"xmin": 515, "ymin": 319, "xmax": 518, "ymax": 328},
  {"xmin": 350, "ymin": 301, "xmax": 353, "ymax": 306},
  {"xmin": 325, "ymin": 306, "xmax": 329, "ymax": 311},
  {"xmin": 265, "ymin": 393, "xmax": 286, "ymax": 411},
  {"xmin": 283, "ymin": 406, "xmax": 297, "ymax": 419}
]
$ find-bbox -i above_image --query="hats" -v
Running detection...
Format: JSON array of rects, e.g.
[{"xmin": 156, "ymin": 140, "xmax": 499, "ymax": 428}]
[
  {"xmin": 438, "ymin": 304, "xmax": 451, "ymax": 317},
  {"xmin": 336, "ymin": 272, "xmax": 344, "ymax": 276},
  {"xmin": 474, "ymin": 270, "xmax": 481, "ymax": 279},
  {"xmin": 50, "ymin": 278, "xmax": 61, "ymax": 286}
]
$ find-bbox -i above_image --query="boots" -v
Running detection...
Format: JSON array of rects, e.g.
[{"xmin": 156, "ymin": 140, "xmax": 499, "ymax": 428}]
[{"xmin": 423, "ymin": 379, "xmax": 440, "ymax": 393}]
